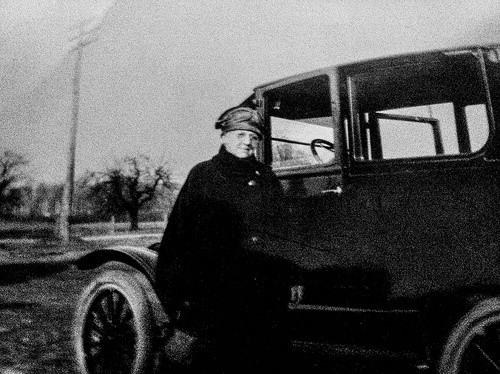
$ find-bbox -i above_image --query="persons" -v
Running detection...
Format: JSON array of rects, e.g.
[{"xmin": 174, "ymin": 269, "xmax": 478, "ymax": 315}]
[{"xmin": 155, "ymin": 106, "xmax": 309, "ymax": 373}]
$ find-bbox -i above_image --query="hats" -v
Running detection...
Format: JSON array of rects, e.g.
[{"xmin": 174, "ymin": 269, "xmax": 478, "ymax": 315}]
[{"xmin": 215, "ymin": 105, "xmax": 264, "ymax": 141}]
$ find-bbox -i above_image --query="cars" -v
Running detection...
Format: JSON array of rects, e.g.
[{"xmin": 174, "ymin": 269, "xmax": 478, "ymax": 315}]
[{"xmin": 71, "ymin": 41, "xmax": 500, "ymax": 374}]
